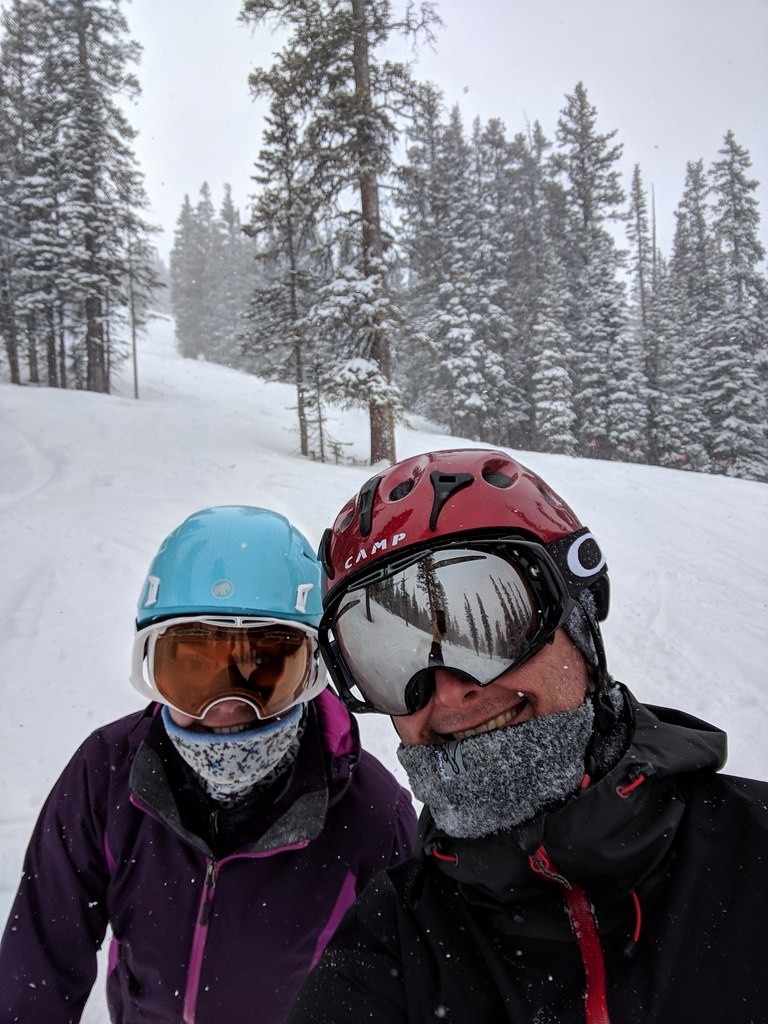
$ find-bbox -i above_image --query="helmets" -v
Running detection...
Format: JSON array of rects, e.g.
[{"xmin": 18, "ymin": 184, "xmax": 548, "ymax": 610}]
[
  {"xmin": 132, "ymin": 505, "xmax": 324, "ymax": 668},
  {"xmin": 317, "ymin": 448, "xmax": 610, "ymax": 713}
]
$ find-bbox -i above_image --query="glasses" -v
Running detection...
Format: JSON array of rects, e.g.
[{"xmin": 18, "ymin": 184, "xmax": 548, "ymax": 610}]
[
  {"xmin": 317, "ymin": 539, "xmax": 574, "ymax": 716},
  {"xmin": 130, "ymin": 615, "xmax": 329, "ymax": 720}
]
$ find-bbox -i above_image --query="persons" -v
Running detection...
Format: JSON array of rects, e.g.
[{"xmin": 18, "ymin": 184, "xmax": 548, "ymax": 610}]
[
  {"xmin": 287, "ymin": 448, "xmax": 767, "ymax": 1023},
  {"xmin": 0, "ymin": 503, "xmax": 422, "ymax": 1023}
]
